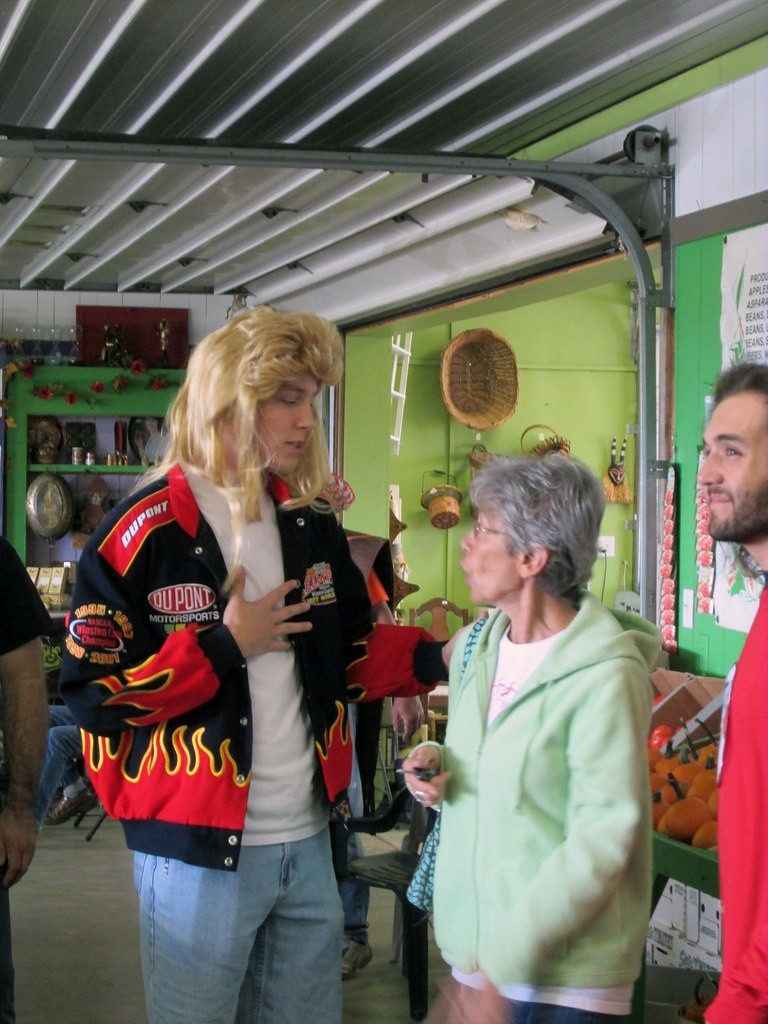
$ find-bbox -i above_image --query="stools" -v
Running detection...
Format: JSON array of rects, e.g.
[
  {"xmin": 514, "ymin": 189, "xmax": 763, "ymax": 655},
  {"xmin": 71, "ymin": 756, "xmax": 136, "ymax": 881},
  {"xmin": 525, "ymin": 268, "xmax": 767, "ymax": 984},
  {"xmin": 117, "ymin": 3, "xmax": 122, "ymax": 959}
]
[{"xmin": 373, "ymin": 723, "xmax": 399, "ymax": 805}]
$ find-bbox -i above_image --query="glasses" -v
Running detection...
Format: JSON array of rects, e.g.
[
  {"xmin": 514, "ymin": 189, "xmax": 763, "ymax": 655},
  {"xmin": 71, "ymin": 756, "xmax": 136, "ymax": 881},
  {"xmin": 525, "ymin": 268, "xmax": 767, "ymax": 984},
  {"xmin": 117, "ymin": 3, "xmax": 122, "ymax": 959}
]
[{"xmin": 473, "ymin": 522, "xmax": 512, "ymax": 540}]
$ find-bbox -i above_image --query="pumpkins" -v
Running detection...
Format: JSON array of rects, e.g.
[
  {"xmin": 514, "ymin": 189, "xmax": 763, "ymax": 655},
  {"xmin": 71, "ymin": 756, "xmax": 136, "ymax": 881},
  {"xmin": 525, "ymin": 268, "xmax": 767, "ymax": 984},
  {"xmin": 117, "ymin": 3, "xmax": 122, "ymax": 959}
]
[{"xmin": 645, "ymin": 718, "xmax": 719, "ymax": 857}]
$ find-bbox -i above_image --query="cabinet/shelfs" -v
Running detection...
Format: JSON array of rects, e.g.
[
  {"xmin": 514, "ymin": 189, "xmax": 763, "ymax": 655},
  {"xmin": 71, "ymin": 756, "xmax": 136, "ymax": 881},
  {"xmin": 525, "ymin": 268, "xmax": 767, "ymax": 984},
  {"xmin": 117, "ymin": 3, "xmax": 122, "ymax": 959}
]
[
  {"xmin": 6, "ymin": 363, "xmax": 189, "ymax": 610},
  {"xmin": 646, "ymin": 733, "xmax": 724, "ymax": 918}
]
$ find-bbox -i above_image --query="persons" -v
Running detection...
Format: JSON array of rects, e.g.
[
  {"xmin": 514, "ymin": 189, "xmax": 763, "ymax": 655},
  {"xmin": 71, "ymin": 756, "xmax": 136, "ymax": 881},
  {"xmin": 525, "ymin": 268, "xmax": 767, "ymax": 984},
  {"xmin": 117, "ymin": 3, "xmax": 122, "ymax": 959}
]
[
  {"xmin": 699, "ymin": 366, "xmax": 768, "ymax": 1024},
  {"xmin": 402, "ymin": 452, "xmax": 663, "ymax": 1024},
  {"xmin": 0, "ymin": 305, "xmax": 489, "ymax": 1024}
]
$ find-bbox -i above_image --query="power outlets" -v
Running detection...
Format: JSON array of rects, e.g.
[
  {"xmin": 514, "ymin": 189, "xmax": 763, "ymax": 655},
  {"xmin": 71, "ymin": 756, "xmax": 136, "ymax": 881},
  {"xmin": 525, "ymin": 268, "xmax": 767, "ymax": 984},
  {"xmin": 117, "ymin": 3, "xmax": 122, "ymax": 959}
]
[{"xmin": 595, "ymin": 535, "xmax": 615, "ymax": 557}]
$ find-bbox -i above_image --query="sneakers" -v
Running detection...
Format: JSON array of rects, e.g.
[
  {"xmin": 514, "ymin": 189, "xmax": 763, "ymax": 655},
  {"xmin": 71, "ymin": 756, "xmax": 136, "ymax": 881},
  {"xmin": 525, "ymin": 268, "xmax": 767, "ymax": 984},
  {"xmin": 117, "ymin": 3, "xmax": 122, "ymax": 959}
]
[{"xmin": 340, "ymin": 936, "xmax": 372, "ymax": 981}]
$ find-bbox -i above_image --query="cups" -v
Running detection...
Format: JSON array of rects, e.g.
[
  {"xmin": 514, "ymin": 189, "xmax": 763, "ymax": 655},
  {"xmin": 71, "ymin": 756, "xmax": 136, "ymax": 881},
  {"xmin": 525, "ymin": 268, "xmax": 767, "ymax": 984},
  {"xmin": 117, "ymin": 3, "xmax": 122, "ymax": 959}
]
[
  {"xmin": 72, "ymin": 447, "xmax": 83, "ymax": 464},
  {"xmin": 85, "ymin": 452, "xmax": 95, "ymax": 465},
  {"xmin": 115, "ymin": 422, "xmax": 127, "ymax": 456}
]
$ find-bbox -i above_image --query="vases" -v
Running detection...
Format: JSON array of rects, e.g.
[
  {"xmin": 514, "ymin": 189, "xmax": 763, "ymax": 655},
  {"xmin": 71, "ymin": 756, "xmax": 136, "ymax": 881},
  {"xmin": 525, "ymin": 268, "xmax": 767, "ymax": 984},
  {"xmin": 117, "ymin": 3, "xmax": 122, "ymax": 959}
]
[{"xmin": 31, "ymin": 441, "xmax": 55, "ymax": 463}]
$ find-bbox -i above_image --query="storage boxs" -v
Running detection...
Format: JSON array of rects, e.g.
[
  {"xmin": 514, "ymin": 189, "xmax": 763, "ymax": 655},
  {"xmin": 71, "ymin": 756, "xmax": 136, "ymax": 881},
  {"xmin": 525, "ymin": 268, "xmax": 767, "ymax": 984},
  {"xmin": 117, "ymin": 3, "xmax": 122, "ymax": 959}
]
[
  {"xmin": 438, "ymin": 328, "xmax": 521, "ymax": 431},
  {"xmin": 649, "ymin": 668, "xmax": 727, "ymax": 754}
]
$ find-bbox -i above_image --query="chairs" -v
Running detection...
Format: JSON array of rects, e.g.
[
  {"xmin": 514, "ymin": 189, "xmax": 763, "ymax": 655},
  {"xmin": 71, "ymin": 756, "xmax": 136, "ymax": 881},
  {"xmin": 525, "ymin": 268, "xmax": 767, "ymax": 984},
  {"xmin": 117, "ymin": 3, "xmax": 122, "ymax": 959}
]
[
  {"xmin": 329, "ymin": 787, "xmax": 439, "ymax": 1021},
  {"xmin": 409, "ymin": 598, "xmax": 469, "ymax": 726}
]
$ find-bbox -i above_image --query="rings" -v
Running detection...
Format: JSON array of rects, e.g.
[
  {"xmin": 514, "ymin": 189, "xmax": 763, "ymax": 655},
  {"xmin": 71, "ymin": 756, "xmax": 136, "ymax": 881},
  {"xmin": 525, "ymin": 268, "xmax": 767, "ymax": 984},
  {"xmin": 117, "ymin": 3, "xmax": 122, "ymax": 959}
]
[{"xmin": 415, "ymin": 791, "xmax": 423, "ymax": 800}]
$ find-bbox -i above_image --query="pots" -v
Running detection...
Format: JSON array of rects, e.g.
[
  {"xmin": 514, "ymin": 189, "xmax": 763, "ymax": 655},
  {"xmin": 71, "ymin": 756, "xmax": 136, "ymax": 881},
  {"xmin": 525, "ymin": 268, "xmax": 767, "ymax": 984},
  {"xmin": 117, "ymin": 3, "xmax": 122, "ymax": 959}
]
[{"xmin": 27, "ymin": 465, "xmax": 72, "ymax": 545}]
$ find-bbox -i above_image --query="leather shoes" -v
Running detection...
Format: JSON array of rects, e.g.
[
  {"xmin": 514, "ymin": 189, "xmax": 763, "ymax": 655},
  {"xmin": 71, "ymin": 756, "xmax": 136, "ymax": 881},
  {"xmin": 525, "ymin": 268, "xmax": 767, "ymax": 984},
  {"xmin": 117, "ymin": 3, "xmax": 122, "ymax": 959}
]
[{"xmin": 43, "ymin": 788, "xmax": 98, "ymax": 825}]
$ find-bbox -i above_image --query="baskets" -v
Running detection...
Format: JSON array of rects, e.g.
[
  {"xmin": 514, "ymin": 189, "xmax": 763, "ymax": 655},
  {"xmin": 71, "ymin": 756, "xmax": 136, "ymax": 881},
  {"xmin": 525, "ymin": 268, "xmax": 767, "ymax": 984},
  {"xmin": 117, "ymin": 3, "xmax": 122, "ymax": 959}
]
[
  {"xmin": 421, "ymin": 470, "xmax": 463, "ymax": 529},
  {"xmin": 469, "ymin": 443, "xmax": 509, "ymax": 469},
  {"xmin": 439, "ymin": 328, "xmax": 519, "ymax": 431},
  {"xmin": 520, "ymin": 424, "xmax": 570, "ymax": 460}
]
